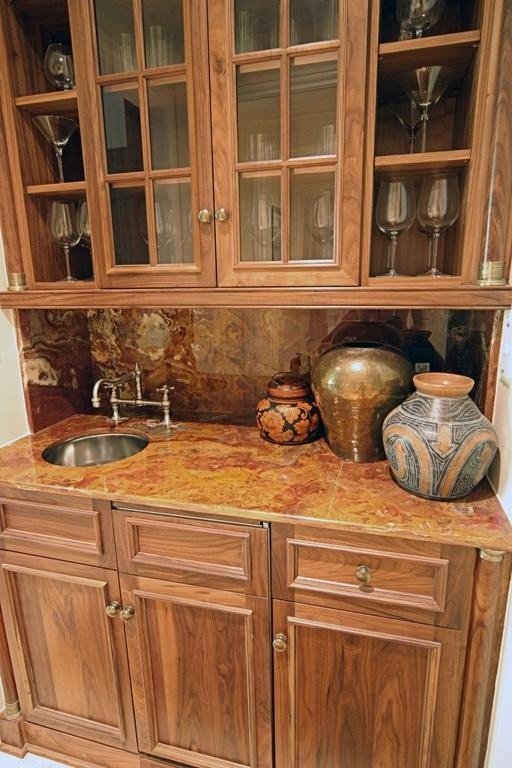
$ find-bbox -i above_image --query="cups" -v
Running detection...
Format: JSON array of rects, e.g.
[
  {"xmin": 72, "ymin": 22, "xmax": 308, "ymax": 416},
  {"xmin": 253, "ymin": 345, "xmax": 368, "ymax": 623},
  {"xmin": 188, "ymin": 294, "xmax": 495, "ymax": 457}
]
[
  {"xmin": 234, "ymin": 0, "xmax": 339, "ymax": 55},
  {"xmin": 110, "ymin": 25, "xmax": 177, "ymax": 74},
  {"xmin": 242, "ymin": 123, "xmax": 339, "ymax": 159}
]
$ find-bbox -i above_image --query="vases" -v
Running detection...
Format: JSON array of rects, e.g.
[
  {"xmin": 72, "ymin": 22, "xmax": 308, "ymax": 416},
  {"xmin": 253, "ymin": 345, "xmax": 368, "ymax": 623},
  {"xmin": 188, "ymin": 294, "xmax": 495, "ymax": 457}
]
[
  {"xmin": 383, "ymin": 370, "xmax": 499, "ymax": 501},
  {"xmin": 313, "ymin": 341, "xmax": 415, "ymax": 463}
]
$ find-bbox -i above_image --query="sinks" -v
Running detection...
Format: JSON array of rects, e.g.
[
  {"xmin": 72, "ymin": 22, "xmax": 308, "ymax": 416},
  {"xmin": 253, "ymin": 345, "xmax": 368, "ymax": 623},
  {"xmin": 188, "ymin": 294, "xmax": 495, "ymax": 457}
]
[{"xmin": 41, "ymin": 427, "xmax": 150, "ymax": 468}]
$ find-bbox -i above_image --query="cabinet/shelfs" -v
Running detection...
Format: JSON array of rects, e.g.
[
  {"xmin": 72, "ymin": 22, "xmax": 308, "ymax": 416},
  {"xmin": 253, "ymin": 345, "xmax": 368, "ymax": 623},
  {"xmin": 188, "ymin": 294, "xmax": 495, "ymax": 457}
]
[{"xmin": 1, "ymin": 0, "xmax": 511, "ymax": 310}]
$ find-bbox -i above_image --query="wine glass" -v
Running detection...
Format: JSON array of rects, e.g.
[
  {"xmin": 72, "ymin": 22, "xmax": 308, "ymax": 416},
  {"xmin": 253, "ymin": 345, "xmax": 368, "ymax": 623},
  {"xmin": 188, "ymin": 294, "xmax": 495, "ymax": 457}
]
[
  {"xmin": 46, "ymin": 198, "xmax": 195, "ymax": 283},
  {"xmin": 29, "ymin": 113, "xmax": 79, "ymax": 183},
  {"xmin": 383, "ymin": 65, "xmax": 458, "ymax": 151},
  {"xmin": 247, "ymin": 188, "xmax": 334, "ymax": 260},
  {"xmin": 417, "ymin": 175, "xmax": 462, "ymax": 280},
  {"xmin": 374, "ymin": 174, "xmax": 413, "ymax": 278},
  {"xmin": 42, "ymin": 38, "xmax": 76, "ymax": 90},
  {"xmin": 393, "ymin": 0, "xmax": 446, "ymax": 42}
]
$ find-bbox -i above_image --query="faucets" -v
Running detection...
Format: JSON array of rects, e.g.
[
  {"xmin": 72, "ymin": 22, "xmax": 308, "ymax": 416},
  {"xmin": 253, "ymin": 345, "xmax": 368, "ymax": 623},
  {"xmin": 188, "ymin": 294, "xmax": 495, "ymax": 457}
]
[{"xmin": 90, "ymin": 371, "xmax": 120, "ymax": 420}]
